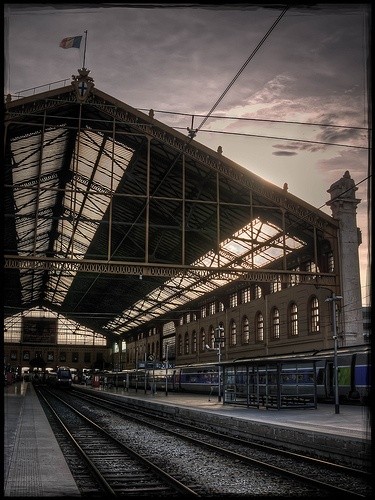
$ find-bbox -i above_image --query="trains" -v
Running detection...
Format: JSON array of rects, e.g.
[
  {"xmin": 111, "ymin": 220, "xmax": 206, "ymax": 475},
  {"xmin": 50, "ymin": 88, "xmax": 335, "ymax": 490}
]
[
  {"xmin": 24, "ymin": 365, "xmax": 72, "ymax": 389},
  {"xmin": 88, "ymin": 342, "xmax": 371, "ymax": 407}
]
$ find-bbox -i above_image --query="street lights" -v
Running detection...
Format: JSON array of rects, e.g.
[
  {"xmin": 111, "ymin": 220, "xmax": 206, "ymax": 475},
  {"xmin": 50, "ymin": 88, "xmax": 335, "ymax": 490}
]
[
  {"xmin": 315, "ymin": 284, "xmax": 343, "ymax": 414},
  {"xmin": 215, "ymin": 325, "xmax": 225, "ymax": 401}
]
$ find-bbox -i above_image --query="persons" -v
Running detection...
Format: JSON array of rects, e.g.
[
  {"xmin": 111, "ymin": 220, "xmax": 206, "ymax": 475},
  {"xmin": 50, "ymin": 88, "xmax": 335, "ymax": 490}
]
[{"xmin": 4, "ymin": 369, "xmax": 176, "ymax": 390}]
